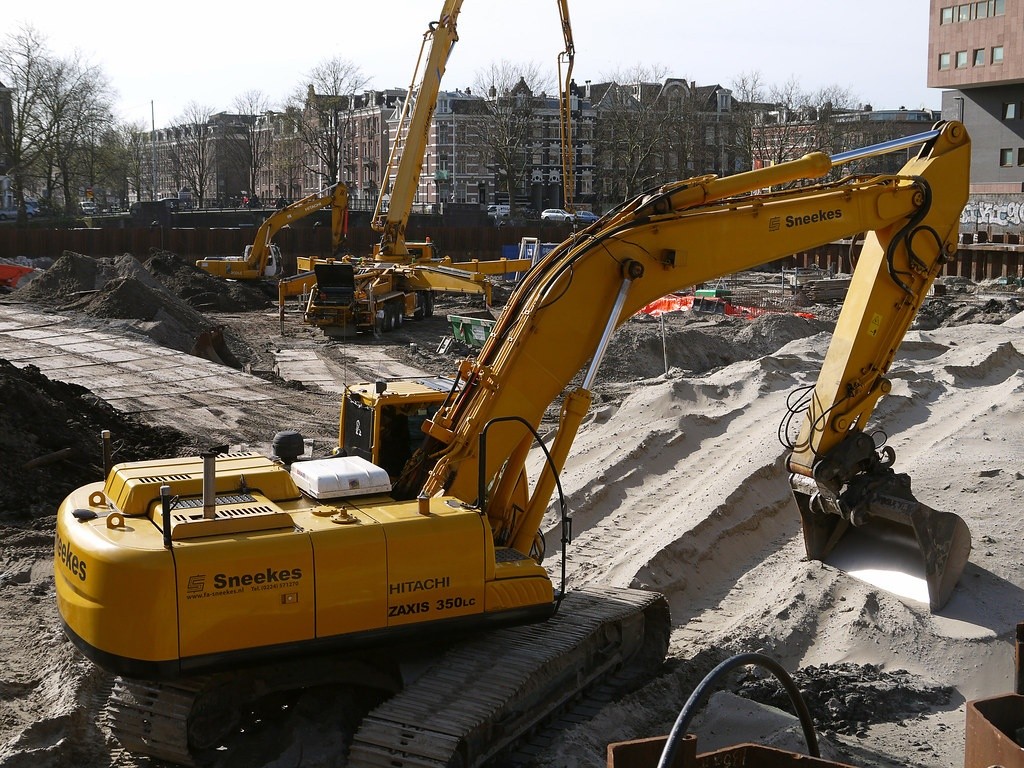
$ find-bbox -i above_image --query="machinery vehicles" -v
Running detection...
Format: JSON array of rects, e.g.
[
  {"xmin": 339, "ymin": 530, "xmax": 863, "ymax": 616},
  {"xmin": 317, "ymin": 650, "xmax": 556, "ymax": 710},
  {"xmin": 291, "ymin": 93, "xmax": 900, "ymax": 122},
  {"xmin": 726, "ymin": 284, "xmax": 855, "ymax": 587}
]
[
  {"xmin": 51, "ymin": 111, "xmax": 972, "ymax": 767},
  {"xmin": 193, "ymin": 181, "xmax": 350, "ymax": 293},
  {"xmin": 277, "ymin": 0, "xmax": 576, "ymax": 342}
]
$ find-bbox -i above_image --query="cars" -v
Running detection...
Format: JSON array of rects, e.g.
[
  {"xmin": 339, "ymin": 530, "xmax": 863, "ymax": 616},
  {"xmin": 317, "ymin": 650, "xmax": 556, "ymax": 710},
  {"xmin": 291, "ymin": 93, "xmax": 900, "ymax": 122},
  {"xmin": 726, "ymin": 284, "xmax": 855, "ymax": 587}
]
[
  {"xmin": 0, "ymin": 203, "xmax": 35, "ymax": 220},
  {"xmin": 574, "ymin": 211, "xmax": 600, "ymax": 224},
  {"xmin": 541, "ymin": 209, "xmax": 575, "ymax": 222},
  {"xmin": 163, "ymin": 198, "xmax": 186, "ymax": 211}
]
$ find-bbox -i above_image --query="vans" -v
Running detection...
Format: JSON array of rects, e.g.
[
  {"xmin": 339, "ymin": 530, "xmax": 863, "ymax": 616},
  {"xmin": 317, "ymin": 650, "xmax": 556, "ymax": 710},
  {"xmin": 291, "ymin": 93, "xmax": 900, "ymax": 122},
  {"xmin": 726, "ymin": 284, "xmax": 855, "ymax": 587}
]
[
  {"xmin": 79, "ymin": 200, "xmax": 95, "ymax": 215},
  {"xmin": 487, "ymin": 205, "xmax": 510, "ymax": 217}
]
